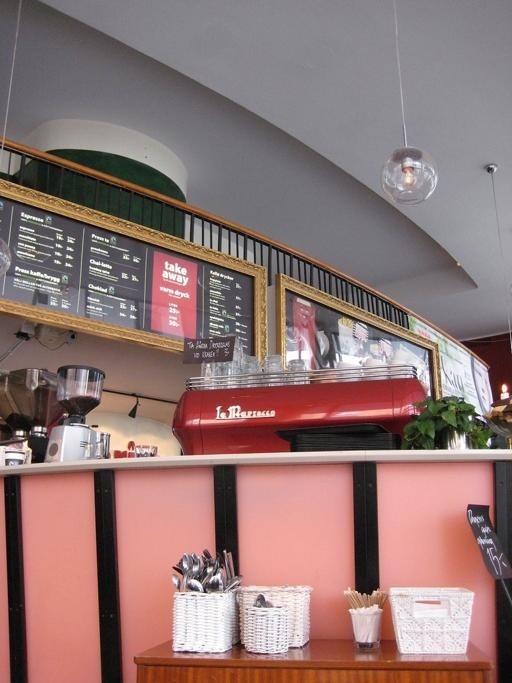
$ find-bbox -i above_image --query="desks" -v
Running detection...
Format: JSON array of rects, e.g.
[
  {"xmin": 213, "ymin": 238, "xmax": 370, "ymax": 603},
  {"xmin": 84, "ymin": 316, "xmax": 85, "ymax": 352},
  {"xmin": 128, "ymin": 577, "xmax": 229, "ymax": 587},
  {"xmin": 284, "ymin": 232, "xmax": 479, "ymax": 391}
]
[{"xmin": 132, "ymin": 638, "xmax": 496, "ymax": 683}]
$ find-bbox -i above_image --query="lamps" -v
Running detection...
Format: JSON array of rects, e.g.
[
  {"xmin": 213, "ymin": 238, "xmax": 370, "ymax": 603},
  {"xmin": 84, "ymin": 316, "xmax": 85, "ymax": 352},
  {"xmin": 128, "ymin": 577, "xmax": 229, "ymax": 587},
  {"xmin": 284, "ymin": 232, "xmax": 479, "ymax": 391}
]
[
  {"xmin": 128, "ymin": 394, "xmax": 141, "ymax": 418},
  {"xmin": 381, "ymin": 0, "xmax": 439, "ymax": 206}
]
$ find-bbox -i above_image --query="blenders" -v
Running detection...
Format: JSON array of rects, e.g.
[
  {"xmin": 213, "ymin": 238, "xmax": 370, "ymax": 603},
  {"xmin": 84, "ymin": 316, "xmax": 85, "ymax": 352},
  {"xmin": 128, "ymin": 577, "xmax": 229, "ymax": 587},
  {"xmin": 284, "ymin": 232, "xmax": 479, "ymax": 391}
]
[{"xmin": 0, "ymin": 363, "xmax": 105, "ymax": 464}]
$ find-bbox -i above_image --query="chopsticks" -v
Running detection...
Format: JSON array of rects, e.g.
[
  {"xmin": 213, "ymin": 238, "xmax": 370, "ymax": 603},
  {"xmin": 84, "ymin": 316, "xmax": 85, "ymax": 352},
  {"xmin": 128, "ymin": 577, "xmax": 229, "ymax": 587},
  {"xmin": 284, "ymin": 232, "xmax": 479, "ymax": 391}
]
[{"xmin": 343, "ymin": 586, "xmax": 387, "ymax": 609}]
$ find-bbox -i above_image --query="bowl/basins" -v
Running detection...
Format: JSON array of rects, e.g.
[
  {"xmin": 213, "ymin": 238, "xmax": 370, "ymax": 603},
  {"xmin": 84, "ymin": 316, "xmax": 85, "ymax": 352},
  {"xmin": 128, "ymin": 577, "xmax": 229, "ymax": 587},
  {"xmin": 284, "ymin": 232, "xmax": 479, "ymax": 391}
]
[{"xmin": 337, "ymin": 359, "xmax": 411, "ymax": 381}]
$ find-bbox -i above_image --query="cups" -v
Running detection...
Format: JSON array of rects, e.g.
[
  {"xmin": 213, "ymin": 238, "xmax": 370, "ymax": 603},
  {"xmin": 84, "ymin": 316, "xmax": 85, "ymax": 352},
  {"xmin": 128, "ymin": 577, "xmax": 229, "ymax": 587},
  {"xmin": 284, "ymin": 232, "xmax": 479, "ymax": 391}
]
[
  {"xmin": 246, "ymin": 355, "xmax": 305, "ymax": 387},
  {"xmin": 348, "ymin": 609, "xmax": 385, "ymax": 649}
]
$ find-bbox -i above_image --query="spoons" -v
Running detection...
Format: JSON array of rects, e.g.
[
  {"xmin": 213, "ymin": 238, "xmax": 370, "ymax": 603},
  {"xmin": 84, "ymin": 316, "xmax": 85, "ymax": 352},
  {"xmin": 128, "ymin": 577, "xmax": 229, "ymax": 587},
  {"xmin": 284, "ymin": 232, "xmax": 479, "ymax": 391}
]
[{"xmin": 171, "ymin": 549, "xmax": 242, "ymax": 593}]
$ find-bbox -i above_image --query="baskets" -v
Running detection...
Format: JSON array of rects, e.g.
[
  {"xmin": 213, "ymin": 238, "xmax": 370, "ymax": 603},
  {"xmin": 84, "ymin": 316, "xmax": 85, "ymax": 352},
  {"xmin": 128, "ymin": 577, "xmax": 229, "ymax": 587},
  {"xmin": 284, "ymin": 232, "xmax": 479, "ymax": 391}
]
[
  {"xmin": 389, "ymin": 587, "xmax": 474, "ymax": 653},
  {"xmin": 171, "ymin": 585, "xmax": 312, "ymax": 654}
]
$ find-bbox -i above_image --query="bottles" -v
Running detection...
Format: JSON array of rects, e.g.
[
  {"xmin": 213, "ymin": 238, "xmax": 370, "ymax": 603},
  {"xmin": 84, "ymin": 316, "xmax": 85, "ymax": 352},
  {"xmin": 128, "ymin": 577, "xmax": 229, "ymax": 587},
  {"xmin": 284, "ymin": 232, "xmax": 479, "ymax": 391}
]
[{"xmin": 201, "ymin": 333, "xmax": 242, "ymax": 389}]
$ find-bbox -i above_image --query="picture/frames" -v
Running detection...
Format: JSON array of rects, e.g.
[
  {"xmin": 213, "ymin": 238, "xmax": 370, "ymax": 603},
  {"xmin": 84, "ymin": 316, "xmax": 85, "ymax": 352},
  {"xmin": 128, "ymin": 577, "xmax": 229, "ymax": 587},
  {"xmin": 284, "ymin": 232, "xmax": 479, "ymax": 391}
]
[
  {"xmin": 0, "ymin": 178, "xmax": 270, "ymax": 370},
  {"xmin": 275, "ymin": 273, "xmax": 442, "ymax": 403}
]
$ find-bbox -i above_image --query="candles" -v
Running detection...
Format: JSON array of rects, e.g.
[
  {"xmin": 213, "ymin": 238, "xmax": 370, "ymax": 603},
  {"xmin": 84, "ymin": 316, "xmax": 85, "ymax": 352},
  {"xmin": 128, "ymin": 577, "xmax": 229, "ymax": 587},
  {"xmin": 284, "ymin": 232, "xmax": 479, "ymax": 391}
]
[{"xmin": 500, "ymin": 383, "xmax": 510, "ymax": 401}]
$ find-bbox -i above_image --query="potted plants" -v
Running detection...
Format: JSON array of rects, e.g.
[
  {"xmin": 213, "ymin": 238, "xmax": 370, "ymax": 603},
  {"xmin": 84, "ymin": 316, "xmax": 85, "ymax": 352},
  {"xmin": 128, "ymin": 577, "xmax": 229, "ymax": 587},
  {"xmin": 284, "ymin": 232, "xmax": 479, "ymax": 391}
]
[{"xmin": 399, "ymin": 396, "xmax": 494, "ymax": 450}]
